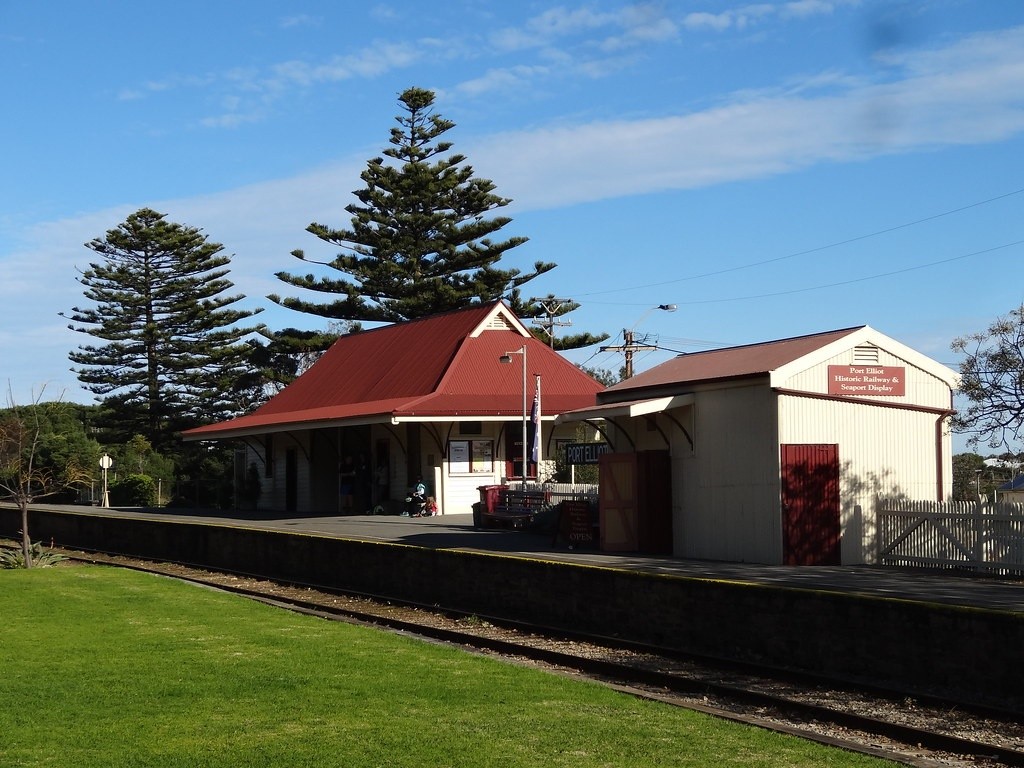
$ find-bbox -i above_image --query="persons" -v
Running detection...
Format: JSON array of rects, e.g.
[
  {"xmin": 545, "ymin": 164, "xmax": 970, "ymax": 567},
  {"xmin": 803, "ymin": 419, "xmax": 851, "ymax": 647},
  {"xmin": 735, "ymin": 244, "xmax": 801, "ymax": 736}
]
[
  {"xmin": 399, "ymin": 474, "xmax": 430, "ymax": 518},
  {"xmin": 375, "ymin": 459, "xmax": 389, "ymax": 508},
  {"xmin": 338, "ymin": 455, "xmax": 356, "ymax": 515}
]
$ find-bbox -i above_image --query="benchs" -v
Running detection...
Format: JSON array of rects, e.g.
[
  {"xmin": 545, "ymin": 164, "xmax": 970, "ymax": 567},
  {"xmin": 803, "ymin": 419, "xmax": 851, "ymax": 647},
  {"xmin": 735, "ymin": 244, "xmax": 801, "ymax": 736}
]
[{"xmin": 481, "ymin": 490, "xmax": 545, "ymax": 531}]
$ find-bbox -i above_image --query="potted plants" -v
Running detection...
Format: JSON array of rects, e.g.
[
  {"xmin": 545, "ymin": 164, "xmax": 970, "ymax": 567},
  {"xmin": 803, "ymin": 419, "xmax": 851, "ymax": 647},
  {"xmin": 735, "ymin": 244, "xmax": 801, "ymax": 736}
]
[{"xmin": 240, "ymin": 462, "xmax": 264, "ymax": 511}]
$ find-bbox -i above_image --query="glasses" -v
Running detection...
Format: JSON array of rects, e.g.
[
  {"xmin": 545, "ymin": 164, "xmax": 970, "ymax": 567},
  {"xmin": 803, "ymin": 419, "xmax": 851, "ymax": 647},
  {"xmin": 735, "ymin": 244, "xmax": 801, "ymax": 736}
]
[{"xmin": 417, "ymin": 476, "xmax": 423, "ymax": 480}]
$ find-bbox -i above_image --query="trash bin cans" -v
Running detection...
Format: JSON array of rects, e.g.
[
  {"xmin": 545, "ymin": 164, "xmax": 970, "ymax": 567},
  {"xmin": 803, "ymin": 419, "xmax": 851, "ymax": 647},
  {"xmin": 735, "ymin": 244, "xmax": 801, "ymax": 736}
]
[
  {"xmin": 472, "ymin": 502, "xmax": 481, "ymax": 528},
  {"xmin": 476, "ymin": 485, "xmax": 510, "ymax": 530}
]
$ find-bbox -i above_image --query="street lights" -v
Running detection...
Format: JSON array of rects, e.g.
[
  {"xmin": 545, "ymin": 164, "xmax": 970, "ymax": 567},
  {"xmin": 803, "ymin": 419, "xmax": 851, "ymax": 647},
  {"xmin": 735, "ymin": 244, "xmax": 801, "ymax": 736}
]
[
  {"xmin": 500, "ymin": 345, "xmax": 528, "ymax": 492},
  {"xmin": 622, "ymin": 302, "xmax": 678, "ymax": 379}
]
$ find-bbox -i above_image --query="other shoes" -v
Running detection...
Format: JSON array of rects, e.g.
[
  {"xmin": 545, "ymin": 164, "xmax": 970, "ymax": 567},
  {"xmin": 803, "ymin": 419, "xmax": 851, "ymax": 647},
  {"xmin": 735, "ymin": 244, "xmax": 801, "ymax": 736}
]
[
  {"xmin": 401, "ymin": 511, "xmax": 409, "ymax": 517},
  {"xmin": 346, "ymin": 507, "xmax": 353, "ymax": 513},
  {"xmin": 343, "ymin": 505, "xmax": 348, "ymax": 510},
  {"xmin": 411, "ymin": 513, "xmax": 421, "ymax": 518}
]
